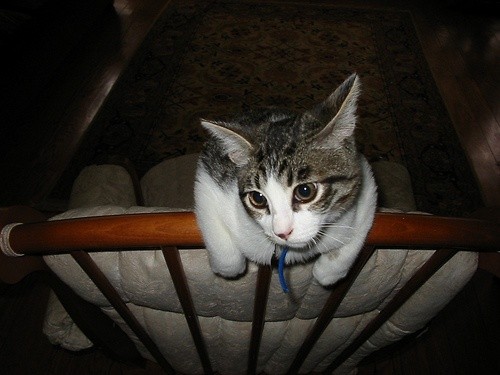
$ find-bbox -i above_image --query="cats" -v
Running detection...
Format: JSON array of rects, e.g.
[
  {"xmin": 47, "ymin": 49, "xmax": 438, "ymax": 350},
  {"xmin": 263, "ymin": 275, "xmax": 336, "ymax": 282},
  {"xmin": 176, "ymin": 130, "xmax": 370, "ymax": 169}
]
[{"xmin": 193, "ymin": 72, "xmax": 379, "ymax": 287}]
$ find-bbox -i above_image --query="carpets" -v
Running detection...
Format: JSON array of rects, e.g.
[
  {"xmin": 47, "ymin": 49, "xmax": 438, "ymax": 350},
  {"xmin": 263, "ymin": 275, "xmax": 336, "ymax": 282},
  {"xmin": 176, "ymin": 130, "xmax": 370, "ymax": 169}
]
[{"xmin": 44, "ymin": 0, "xmax": 488, "ymax": 218}]
